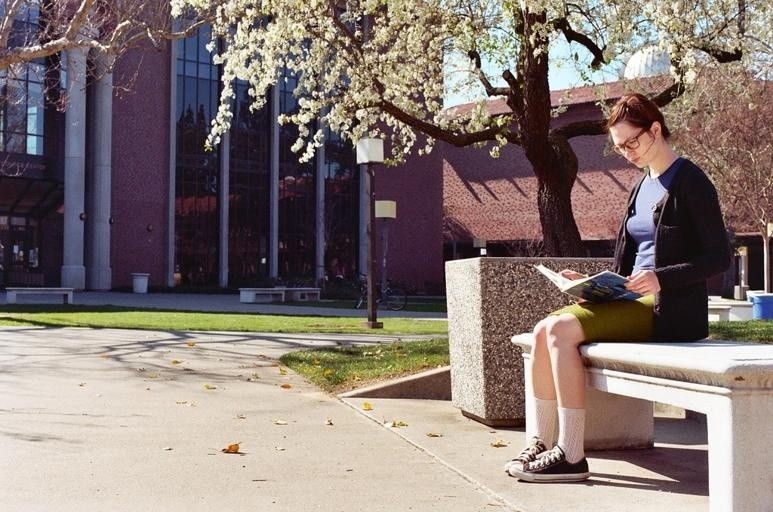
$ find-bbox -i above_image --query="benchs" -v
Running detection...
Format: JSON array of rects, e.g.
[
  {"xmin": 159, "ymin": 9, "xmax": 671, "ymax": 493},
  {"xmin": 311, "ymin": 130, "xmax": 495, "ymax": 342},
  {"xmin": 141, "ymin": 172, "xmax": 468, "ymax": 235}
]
[
  {"xmin": 238, "ymin": 286, "xmax": 322, "ymax": 304},
  {"xmin": 5, "ymin": 286, "xmax": 76, "ymax": 305},
  {"xmin": 508, "ymin": 331, "xmax": 772, "ymax": 512},
  {"xmin": 707, "ymin": 295, "xmax": 753, "ymax": 321}
]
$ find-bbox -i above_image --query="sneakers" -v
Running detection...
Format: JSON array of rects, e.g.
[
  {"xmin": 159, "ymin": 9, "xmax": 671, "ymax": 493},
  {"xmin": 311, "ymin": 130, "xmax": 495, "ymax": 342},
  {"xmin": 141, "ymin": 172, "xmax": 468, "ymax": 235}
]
[{"xmin": 505, "ymin": 436, "xmax": 591, "ymax": 483}]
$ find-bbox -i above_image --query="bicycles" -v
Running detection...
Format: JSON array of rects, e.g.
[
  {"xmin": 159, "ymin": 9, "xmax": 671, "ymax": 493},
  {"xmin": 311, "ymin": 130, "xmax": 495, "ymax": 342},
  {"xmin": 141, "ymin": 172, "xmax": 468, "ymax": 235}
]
[{"xmin": 352, "ymin": 272, "xmax": 408, "ymax": 312}]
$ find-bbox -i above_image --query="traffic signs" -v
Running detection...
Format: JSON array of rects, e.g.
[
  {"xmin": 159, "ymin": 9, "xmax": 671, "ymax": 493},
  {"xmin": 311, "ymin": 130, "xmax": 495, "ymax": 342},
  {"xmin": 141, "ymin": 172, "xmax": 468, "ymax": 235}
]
[{"xmin": 352, "ymin": 135, "xmax": 387, "ymax": 328}]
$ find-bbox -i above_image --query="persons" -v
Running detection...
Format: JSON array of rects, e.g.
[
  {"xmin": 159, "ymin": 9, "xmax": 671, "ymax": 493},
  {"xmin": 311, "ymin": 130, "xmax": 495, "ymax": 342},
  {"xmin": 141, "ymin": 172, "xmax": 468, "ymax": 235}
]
[{"xmin": 507, "ymin": 93, "xmax": 731, "ymax": 482}]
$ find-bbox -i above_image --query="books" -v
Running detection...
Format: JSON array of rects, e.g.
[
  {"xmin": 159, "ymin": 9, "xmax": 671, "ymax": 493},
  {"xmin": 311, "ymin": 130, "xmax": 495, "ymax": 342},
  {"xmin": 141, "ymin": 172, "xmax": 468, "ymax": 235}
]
[{"xmin": 535, "ymin": 264, "xmax": 641, "ymax": 304}]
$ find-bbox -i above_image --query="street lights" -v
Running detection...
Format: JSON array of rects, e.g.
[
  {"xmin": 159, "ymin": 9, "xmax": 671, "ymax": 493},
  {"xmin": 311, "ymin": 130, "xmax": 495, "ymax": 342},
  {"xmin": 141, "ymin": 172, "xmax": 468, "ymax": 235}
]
[{"xmin": 371, "ymin": 199, "xmax": 397, "ymax": 303}]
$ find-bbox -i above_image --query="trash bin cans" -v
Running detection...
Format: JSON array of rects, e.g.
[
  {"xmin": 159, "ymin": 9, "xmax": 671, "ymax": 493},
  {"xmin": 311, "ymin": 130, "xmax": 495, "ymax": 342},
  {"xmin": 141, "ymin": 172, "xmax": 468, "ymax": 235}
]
[
  {"xmin": 749, "ymin": 293, "xmax": 773, "ymax": 321},
  {"xmin": 130, "ymin": 272, "xmax": 151, "ymax": 294}
]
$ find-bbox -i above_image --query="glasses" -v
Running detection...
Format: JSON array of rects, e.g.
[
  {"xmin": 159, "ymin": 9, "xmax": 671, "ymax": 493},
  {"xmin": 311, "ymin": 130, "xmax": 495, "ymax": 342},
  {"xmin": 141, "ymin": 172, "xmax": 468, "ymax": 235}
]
[{"xmin": 613, "ymin": 128, "xmax": 649, "ymax": 155}]
